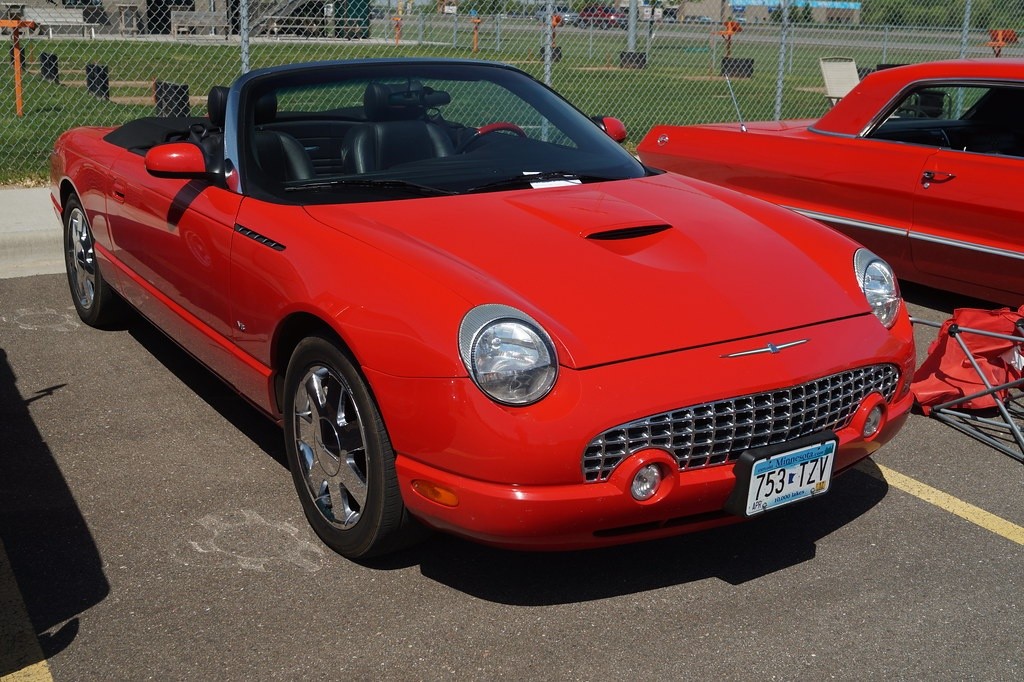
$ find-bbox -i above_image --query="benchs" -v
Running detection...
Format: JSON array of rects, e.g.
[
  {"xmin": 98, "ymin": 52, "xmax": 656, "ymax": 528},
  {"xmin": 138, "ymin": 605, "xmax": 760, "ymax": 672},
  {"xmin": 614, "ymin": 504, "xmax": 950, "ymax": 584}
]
[{"xmin": 870, "ymin": 118, "xmax": 1008, "ymax": 152}]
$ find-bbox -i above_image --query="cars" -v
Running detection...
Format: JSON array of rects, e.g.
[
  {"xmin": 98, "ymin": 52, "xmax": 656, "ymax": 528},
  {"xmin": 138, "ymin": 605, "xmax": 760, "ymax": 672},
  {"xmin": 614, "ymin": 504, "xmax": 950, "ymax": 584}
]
[{"xmin": 637, "ymin": 58, "xmax": 1024, "ymax": 305}]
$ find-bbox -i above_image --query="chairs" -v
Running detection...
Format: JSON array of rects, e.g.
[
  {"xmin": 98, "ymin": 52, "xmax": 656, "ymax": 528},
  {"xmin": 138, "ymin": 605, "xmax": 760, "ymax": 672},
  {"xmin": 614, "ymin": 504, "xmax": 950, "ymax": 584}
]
[
  {"xmin": 911, "ymin": 303, "xmax": 1024, "ymax": 463},
  {"xmin": 342, "ymin": 80, "xmax": 455, "ymax": 172},
  {"xmin": 201, "ymin": 85, "xmax": 318, "ymax": 181}
]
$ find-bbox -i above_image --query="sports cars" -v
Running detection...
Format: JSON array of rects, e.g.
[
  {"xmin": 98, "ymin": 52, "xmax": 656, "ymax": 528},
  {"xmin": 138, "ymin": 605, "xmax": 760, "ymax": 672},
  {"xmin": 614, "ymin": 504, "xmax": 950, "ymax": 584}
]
[{"xmin": 49, "ymin": 57, "xmax": 917, "ymax": 557}]
